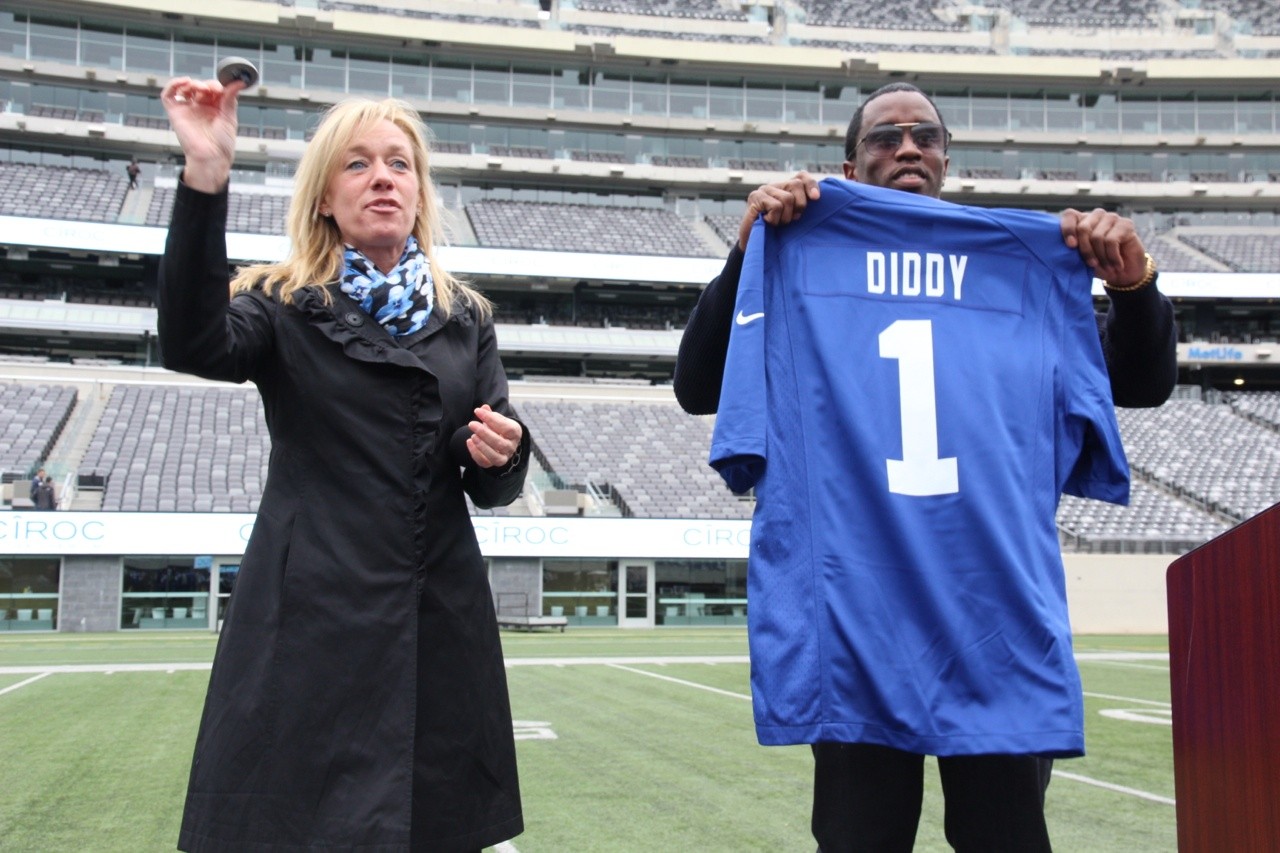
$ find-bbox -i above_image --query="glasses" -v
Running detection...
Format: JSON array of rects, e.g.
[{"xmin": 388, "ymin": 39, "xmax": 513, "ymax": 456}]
[{"xmin": 846, "ymin": 122, "xmax": 952, "ymax": 159}]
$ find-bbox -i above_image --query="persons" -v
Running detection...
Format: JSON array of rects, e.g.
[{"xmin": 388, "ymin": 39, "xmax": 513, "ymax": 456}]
[
  {"xmin": 30, "ymin": 469, "xmax": 56, "ymax": 510},
  {"xmin": 127, "ymin": 162, "xmax": 140, "ymax": 188},
  {"xmin": 157, "ymin": 77, "xmax": 533, "ymax": 853},
  {"xmin": 674, "ymin": 81, "xmax": 1178, "ymax": 853}
]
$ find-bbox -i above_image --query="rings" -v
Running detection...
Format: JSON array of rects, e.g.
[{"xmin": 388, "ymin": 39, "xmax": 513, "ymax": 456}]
[{"xmin": 176, "ymin": 96, "xmax": 185, "ymax": 101}]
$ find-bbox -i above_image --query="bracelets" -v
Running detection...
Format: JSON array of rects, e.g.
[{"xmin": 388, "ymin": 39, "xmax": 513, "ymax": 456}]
[{"xmin": 1103, "ymin": 253, "xmax": 1155, "ymax": 290}]
